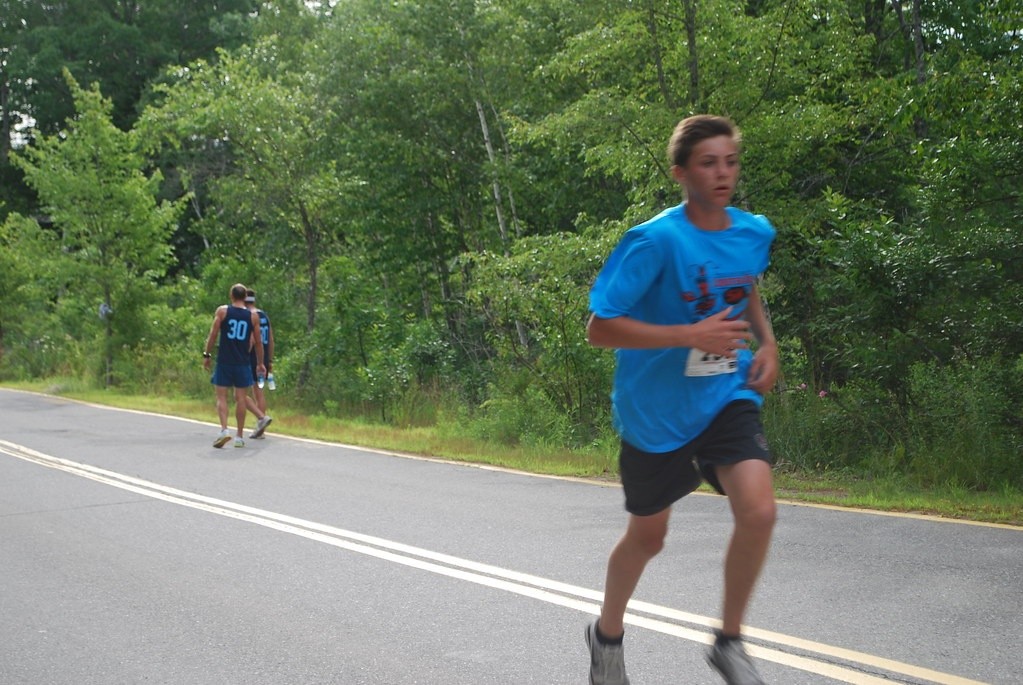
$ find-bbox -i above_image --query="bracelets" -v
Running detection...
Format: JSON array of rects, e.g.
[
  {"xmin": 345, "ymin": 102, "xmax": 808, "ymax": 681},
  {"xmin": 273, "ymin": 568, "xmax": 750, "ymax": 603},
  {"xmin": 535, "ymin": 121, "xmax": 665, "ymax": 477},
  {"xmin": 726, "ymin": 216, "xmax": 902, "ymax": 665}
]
[
  {"xmin": 203, "ymin": 352, "xmax": 212, "ymax": 358},
  {"xmin": 268, "ymin": 359, "xmax": 274, "ymax": 363}
]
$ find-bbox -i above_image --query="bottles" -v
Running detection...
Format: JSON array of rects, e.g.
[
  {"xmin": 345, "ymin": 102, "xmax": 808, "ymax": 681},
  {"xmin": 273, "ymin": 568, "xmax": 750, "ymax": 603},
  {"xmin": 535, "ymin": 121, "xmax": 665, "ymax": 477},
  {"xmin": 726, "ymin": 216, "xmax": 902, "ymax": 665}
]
[
  {"xmin": 257, "ymin": 374, "xmax": 264, "ymax": 388},
  {"xmin": 267, "ymin": 372, "xmax": 276, "ymax": 391}
]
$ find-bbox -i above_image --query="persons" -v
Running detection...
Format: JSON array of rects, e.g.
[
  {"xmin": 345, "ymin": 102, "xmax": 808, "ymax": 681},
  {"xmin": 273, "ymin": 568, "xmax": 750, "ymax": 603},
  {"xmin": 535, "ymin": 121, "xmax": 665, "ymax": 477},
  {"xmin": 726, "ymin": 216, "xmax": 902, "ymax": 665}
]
[
  {"xmin": 583, "ymin": 112, "xmax": 781, "ymax": 685},
  {"xmin": 202, "ymin": 282, "xmax": 266, "ymax": 447},
  {"xmin": 244, "ymin": 288, "xmax": 275, "ymax": 439}
]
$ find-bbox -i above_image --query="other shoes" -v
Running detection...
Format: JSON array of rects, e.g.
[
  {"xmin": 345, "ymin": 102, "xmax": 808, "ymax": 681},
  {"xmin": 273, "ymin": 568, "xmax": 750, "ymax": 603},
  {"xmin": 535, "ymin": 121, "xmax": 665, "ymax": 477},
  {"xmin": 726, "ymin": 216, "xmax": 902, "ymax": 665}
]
[
  {"xmin": 584, "ymin": 618, "xmax": 630, "ymax": 685},
  {"xmin": 253, "ymin": 415, "xmax": 272, "ymax": 436},
  {"xmin": 233, "ymin": 437, "xmax": 245, "ymax": 448},
  {"xmin": 212, "ymin": 428, "xmax": 233, "ymax": 448},
  {"xmin": 249, "ymin": 431, "xmax": 266, "ymax": 439},
  {"xmin": 705, "ymin": 631, "xmax": 766, "ymax": 685}
]
